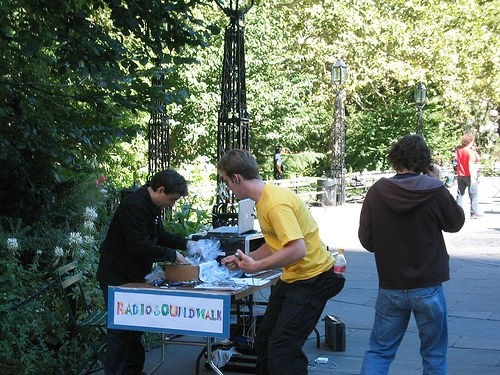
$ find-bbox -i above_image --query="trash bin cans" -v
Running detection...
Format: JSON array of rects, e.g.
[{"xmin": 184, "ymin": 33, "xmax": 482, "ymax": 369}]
[{"xmin": 317, "ymin": 180, "xmax": 336, "ymax": 206}]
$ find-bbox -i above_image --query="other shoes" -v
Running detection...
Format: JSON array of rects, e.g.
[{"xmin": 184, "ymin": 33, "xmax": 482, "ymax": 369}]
[{"xmin": 471, "ymin": 214, "xmax": 481, "ymax": 218}]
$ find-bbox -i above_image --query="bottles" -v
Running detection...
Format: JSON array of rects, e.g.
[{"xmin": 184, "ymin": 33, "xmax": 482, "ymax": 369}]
[{"xmin": 333, "ymin": 248, "xmax": 347, "ymax": 278}]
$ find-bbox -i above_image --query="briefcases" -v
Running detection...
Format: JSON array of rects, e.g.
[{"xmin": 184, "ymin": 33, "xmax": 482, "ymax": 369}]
[{"xmin": 322, "ymin": 314, "xmax": 346, "ymax": 351}]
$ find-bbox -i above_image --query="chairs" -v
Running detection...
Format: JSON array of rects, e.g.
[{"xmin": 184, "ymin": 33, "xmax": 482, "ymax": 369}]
[{"xmin": 59, "ymin": 261, "xmax": 110, "ymax": 375}]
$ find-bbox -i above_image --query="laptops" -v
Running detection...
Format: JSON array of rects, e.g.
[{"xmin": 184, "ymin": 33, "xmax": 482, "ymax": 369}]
[{"xmin": 207, "ymin": 198, "xmax": 255, "ymax": 234}]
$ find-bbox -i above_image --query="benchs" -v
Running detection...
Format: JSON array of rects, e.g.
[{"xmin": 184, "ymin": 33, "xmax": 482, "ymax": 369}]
[
  {"xmin": 267, "ymin": 177, "xmax": 329, "ymax": 208},
  {"xmin": 437, "ymin": 161, "xmax": 500, "ymax": 178},
  {"xmin": 346, "ymin": 171, "xmax": 397, "ymax": 201}
]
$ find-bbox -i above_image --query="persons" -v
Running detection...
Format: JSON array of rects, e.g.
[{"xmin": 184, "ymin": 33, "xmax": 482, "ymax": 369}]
[
  {"xmin": 274, "ymin": 146, "xmax": 285, "ymax": 181},
  {"xmin": 217, "ymin": 148, "xmax": 335, "ymax": 375},
  {"xmin": 431, "ymin": 142, "xmax": 480, "ymax": 187},
  {"xmin": 358, "ymin": 134, "xmax": 466, "ymax": 375},
  {"xmin": 96, "ymin": 169, "xmax": 203, "ymax": 375},
  {"xmin": 456, "ymin": 133, "xmax": 483, "ymax": 219}
]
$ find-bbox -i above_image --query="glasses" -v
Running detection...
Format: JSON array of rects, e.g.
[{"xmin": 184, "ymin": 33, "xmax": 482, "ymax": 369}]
[{"xmin": 223, "ymin": 175, "xmax": 233, "ymax": 185}]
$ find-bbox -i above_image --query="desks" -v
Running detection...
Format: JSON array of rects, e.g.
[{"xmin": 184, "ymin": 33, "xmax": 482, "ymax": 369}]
[{"xmin": 117, "ymin": 270, "xmax": 321, "ymax": 375}]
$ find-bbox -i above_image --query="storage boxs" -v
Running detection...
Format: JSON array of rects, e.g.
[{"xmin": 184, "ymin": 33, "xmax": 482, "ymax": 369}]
[
  {"xmin": 164, "ymin": 260, "xmax": 220, "ymax": 284},
  {"xmin": 321, "ymin": 315, "xmax": 346, "ymax": 350}
]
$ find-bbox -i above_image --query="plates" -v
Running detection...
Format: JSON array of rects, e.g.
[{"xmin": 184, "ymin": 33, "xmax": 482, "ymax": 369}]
[{"xmin": 194, "ymin": 281, "xmax": 248, "ymax": 291}]
